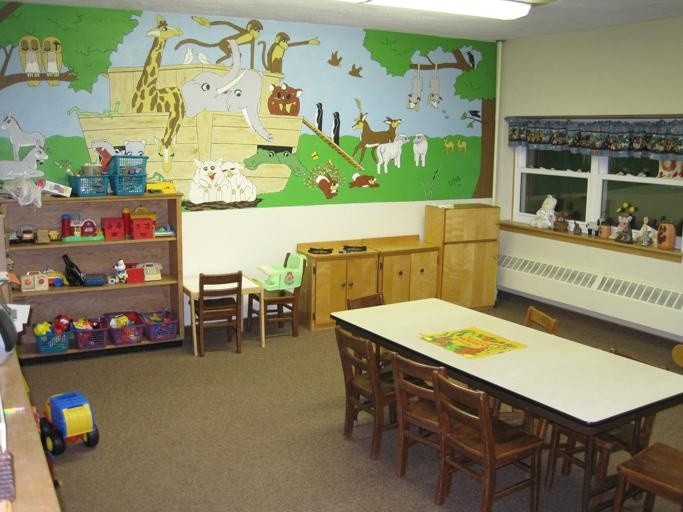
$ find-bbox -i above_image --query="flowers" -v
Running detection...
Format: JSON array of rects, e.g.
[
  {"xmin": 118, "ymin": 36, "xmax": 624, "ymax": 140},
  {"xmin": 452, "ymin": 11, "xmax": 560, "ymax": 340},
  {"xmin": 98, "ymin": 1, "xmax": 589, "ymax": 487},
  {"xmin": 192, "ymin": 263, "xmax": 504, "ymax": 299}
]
[{"xmin": 616, "ymin": 202, "xmax": 637, "ymax": 217}]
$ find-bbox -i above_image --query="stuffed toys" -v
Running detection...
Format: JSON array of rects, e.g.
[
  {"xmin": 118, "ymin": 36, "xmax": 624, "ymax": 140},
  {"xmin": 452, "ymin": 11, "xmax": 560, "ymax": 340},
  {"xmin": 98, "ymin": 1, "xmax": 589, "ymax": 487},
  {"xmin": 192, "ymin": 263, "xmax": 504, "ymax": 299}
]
[
  {"xmin": 552, "ymin": 211, "xmax": 568, "ymax": 232},
  {"xmin": 530, "ymin": 194, "xmax": 557, "ymax": 230}
]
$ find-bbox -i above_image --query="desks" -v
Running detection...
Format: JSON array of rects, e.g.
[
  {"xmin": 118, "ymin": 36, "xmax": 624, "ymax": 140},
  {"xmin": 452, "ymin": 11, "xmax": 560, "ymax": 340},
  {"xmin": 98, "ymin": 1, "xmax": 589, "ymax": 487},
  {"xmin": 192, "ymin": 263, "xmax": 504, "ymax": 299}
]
[
  {"xmin": 330, "ymin": 297, "xmax": 682, "ymax": 512},
  {"xmin": 0, "ymin": 348, "xmax": 65, "ymax": 512},
  {"xmin": 183, "ymin": 274, "xmax": 266, "ymax": 356}
]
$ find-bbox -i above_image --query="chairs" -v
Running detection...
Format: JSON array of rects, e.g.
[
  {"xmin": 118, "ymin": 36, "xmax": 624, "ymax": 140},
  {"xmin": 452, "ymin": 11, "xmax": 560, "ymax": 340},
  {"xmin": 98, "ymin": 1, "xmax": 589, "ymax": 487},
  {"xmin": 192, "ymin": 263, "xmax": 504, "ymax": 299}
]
[
  {"xmin": 543, "ymin": 347, "xmax": 670, "ymax": 512},
  {"xmin": 431, "ymin": 370, "xmax": 543, "ymax": 512},
  {"xmin": 334, "ymin": 324, "xmax": 426, "ymax": 462},
  {"xmin": 247, "ymin": 252, "xmax": 308, "ymax": 342},
  {"xmin": 612, "ymin": 441, "xmax": 683, "ymax": 512},
  {"xmin": 487, "ymin": 305, "xmax": 560, "ymax": 432},
  {"xmin": 189, "ymin": 271, "xmax": 242, "ymax": 357},
  {"xmin": 347, "ymin": 291, "xmax": 398, "ymax": 426},
  {"xmin": 390, "ymin": 351, "xmax": 494, "ymax": 495}
]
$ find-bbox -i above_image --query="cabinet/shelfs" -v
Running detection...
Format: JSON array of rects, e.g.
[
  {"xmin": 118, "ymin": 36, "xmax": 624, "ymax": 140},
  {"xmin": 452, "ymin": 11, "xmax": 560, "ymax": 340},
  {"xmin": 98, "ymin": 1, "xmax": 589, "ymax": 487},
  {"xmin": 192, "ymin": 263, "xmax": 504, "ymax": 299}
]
[
  {"xmin": 362, "ymin": 234, "xmax": 440, "ymax": 304},
  {"xmin": 424, "ymin": 203, "xmax": 500, "ymax": 310},
  {"xmin": 0, "ymin": 193, "xmax": 184, "ymax": 361},
  {"xmin": 294, "ymin": 239, "xmax": 380, "ymax": 334}
]
